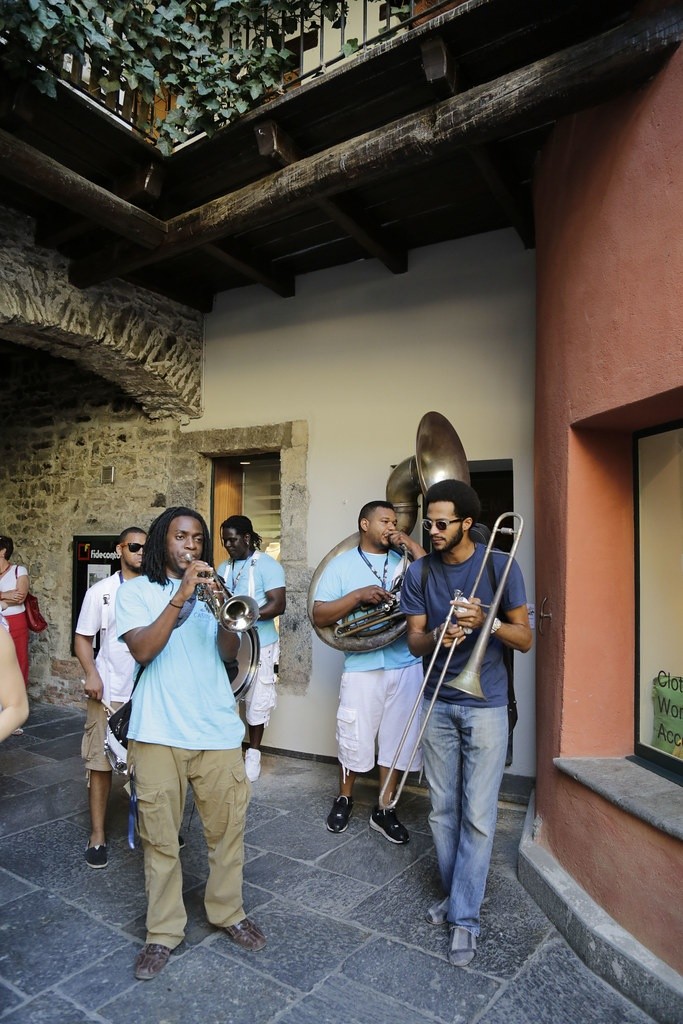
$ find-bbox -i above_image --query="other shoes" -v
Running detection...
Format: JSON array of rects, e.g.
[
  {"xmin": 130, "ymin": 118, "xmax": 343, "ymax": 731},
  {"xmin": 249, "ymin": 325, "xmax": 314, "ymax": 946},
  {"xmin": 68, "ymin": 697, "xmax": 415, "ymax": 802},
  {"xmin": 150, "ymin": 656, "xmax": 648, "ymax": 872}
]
[
  {"xmin": 425, "ymin": 895, "xmax": 450, "ymax": 925},
  {"xmin": 83, "ymin": 837, "xmax": 108, "ymax": 868},
  {"xmin": 447, "ymin": 927, "xmax": 477, "ymax": 966},
  {"xmin": 11, "ymin": 728, "xmax": 24, "ymax": 735},
  {"xmin": 178, "ymin": 834, "xmax": 187, "ymax": 849}
]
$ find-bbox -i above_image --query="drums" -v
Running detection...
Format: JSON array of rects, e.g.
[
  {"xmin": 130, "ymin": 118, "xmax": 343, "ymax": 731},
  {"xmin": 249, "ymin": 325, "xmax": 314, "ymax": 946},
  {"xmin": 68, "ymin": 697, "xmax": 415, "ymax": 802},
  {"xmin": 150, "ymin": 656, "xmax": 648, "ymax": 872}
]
[
  {"xmin": 217, "ymin": 626, "xmax": 261, "ymax": 702},
  {"xmin": 104, "ymin": 699, "xmax": 133, "ymax": 776}
]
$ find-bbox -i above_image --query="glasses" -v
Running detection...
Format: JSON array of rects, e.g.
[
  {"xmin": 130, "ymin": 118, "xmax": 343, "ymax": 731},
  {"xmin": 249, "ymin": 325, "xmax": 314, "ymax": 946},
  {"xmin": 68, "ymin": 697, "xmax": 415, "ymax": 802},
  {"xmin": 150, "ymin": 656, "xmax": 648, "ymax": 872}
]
[
  {"xmin": 421, "ymin": 517, "xmax": 465, "ymax": 531},
  {"xmin": 120, "ymin": 542, "xmax": 145, "ymax": 553}
]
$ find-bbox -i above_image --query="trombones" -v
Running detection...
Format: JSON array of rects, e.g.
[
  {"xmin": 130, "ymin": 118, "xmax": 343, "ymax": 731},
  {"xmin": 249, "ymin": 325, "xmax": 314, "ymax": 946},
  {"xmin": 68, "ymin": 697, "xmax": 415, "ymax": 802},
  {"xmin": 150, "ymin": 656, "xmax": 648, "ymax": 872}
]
[{"xmin": 377, "ymin": 512, "xmax": 524, "ymax": 811}]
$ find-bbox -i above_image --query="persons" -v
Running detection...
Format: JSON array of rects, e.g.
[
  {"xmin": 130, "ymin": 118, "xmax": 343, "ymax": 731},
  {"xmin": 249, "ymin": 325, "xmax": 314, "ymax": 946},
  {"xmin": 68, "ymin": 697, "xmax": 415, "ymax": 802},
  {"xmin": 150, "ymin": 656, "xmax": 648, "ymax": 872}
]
[
  {"xmin": 75, "ymin": 527, "xmax": 186, "ymax": 868},
  {"xmin": 400, "ymin": 478, "xmax": 533, "ymax": 965},
  {"xmin": 0, "ymin": 534, "xmax": 30, "ymax": 685},
  {"xmin": 312, "ymin": 501, "xmax": 426, "ymax": 844},
  {"xmin": 115, "ymin": 506, "xmax": 266, "ymax": 979},
  {"xmin": 217, "ymin": 515, "xmax": 285, "ymax": 782},
  {"xmin": 0, "ymin": 625, "xmax": 30, "ymax": 743}
]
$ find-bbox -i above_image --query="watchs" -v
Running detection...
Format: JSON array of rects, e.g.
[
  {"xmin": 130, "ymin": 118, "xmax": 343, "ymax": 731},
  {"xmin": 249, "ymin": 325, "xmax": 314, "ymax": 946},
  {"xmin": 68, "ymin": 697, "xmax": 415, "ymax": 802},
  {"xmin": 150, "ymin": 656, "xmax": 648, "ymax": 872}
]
[{"xmin": 491, "ymin": 618, "xmax": 501, "ymax": 634}]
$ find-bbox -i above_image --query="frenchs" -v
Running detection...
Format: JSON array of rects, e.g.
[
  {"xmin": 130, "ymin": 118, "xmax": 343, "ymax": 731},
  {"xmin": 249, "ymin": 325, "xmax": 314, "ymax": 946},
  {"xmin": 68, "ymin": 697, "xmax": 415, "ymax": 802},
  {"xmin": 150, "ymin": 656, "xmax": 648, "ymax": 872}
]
[{"xmin": 306, "ymin": 411, "xmax": 471, "ymax": 653}]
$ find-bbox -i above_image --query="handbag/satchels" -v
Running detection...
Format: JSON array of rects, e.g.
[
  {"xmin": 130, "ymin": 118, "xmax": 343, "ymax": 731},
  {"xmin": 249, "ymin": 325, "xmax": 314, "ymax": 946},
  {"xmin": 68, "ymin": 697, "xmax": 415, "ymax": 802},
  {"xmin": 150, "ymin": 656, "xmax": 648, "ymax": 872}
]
[{"xmin": 15, "ymin": 564, "xmax": 48, "ymax": 633}]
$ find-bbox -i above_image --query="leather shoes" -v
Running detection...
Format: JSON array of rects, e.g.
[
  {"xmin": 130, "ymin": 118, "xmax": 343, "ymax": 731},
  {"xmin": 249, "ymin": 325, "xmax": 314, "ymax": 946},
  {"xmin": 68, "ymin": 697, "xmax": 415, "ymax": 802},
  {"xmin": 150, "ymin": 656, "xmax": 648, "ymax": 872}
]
[
  {"xmin": 135, "ymin": 944, "xmax": 170, "ymax": 979},
  {"xmin": 225, "ymin": 917, "xmax": 267, "ymax": 951}
]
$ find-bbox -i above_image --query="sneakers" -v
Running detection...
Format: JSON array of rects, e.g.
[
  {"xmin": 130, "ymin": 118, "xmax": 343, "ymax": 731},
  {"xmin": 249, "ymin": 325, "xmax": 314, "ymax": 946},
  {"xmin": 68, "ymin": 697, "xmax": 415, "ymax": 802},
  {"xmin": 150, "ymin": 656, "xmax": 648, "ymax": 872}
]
[
  {"xmin": 326, "ymin": 795, "xmax": 354, "ymax": 833},
  {"xmin": 368, "ymin": 804, "xmax": 411, "ymax": 843},
  {"xmin": 245, "ymin": 748, "xmax": 262, "ymax": 782}
]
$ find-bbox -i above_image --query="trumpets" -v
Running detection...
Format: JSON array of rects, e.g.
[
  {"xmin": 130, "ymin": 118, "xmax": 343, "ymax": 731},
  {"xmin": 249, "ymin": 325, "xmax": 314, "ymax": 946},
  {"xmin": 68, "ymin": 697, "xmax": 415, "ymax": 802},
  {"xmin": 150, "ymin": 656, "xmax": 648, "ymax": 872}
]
[{"xmin": 182, "ymin": 552, "xmax": 260, "ymax": 633}]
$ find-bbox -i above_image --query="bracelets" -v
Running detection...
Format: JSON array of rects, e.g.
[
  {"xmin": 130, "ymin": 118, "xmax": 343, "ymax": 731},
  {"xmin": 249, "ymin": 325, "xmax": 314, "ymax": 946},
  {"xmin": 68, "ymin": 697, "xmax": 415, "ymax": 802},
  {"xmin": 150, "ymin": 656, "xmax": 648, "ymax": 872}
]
[
  {"xmin": 434, "ymin": 627, "xmax": 438, "ymax": 645},
  {"xmin": 169, "ymin": 600, "xmax": 183, "ymax": 608}
]
